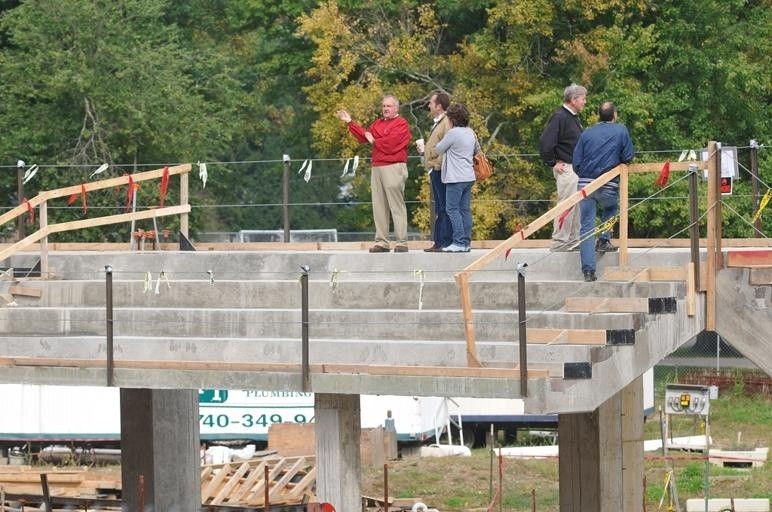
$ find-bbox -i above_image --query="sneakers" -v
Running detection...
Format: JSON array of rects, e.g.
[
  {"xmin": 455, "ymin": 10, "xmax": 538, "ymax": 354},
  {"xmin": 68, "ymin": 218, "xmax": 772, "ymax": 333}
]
[
  {"xmin": 369, "ymin": 245, "xmax": 390, "ymax": 252},
  {"xmin": 550, "ymin": 241, "xmax": 581, "ymax": 252},
  {"xmin": 595, "ymin": 239, "xmax": 619, "ymax": 252},
  {"xmin": 424, "ymin": 244, "xmax": 443, "ymax": 252},
  {"xmin": 442, "ymin": 244, "xmax": 471, "ymax": 253},
  {"xmin": 584, "ymin": 271, "xmax": 597, "ymax": 282},
  {"xmin": 394, "ymin": 246, "xmax": 408, "ymax": 252}
]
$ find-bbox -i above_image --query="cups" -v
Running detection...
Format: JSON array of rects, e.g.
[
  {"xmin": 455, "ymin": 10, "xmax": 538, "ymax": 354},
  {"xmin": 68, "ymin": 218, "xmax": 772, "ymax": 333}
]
[{"xmin": 416, "ymin": 139, "xmax": 425, "ymax": 152}]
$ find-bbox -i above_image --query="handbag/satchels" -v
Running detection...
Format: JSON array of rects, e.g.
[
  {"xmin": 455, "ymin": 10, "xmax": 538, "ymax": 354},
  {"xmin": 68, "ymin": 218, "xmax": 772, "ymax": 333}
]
[{"xmin": 473, "ymin": 151, "xmax": 493, "ymax": 180}]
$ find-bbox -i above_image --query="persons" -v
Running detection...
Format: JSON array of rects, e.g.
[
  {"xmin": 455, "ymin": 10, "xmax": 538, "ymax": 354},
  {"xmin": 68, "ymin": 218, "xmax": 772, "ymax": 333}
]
[
  {"xmin": 334, "ymin": 94, "xmax": 412, "ymax": 252},
  {"xmin": 571, "ymin": 101, "xmax": 632, "ymax": 283},
  {"xmin": 414, "ymin": 90, "xmax": 456, "ymax": 254},
  {"xmin": 538, "ymin": 82, "xmax": 589, "ymax": 252},
  {"xmin": 433, "ymin": 101, "xmax": 481, "ymax": 254}
]
